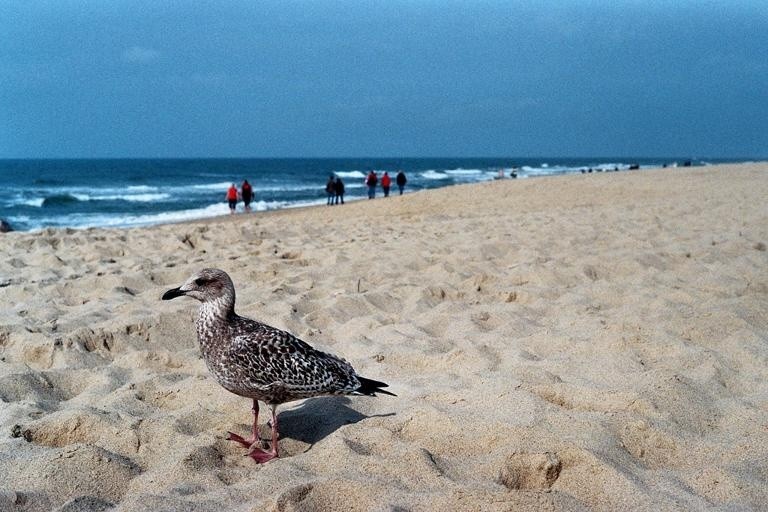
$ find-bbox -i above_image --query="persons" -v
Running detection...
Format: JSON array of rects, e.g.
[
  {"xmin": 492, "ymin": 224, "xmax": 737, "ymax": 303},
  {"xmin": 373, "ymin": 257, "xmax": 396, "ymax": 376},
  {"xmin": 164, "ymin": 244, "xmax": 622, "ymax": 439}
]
[
  {"xmin": 326, "ymin": 176, "xmax": 336, "ymax": 205},
  {"xmin": 335, "ymin": 178, "xmax": 344, "ymax": 204},
  {"xmin": 367, "ymin": 170, "xmax": 377, "ymax": 199},
  {"xmin": 242, "ymin": 179, "xmax": 251, "ymax": 207},
  {"xmin": 396, "ymin": 170, "xmax": 406, "ymax": 194},
  {"xmin": 224, "ymin": 184, "xmax": 238, "ymax": 212},
  {"xmin": 383, "ymin": 172, "xmax": 390, "ymax": 197}
]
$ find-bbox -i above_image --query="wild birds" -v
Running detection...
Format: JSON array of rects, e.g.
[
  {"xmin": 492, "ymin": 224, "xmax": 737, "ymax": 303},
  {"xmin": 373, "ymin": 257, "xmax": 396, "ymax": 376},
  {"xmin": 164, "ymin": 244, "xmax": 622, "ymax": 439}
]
[{"xmin": 162, "ymin": 268, "xmax": 397, "ymax": 462}]
